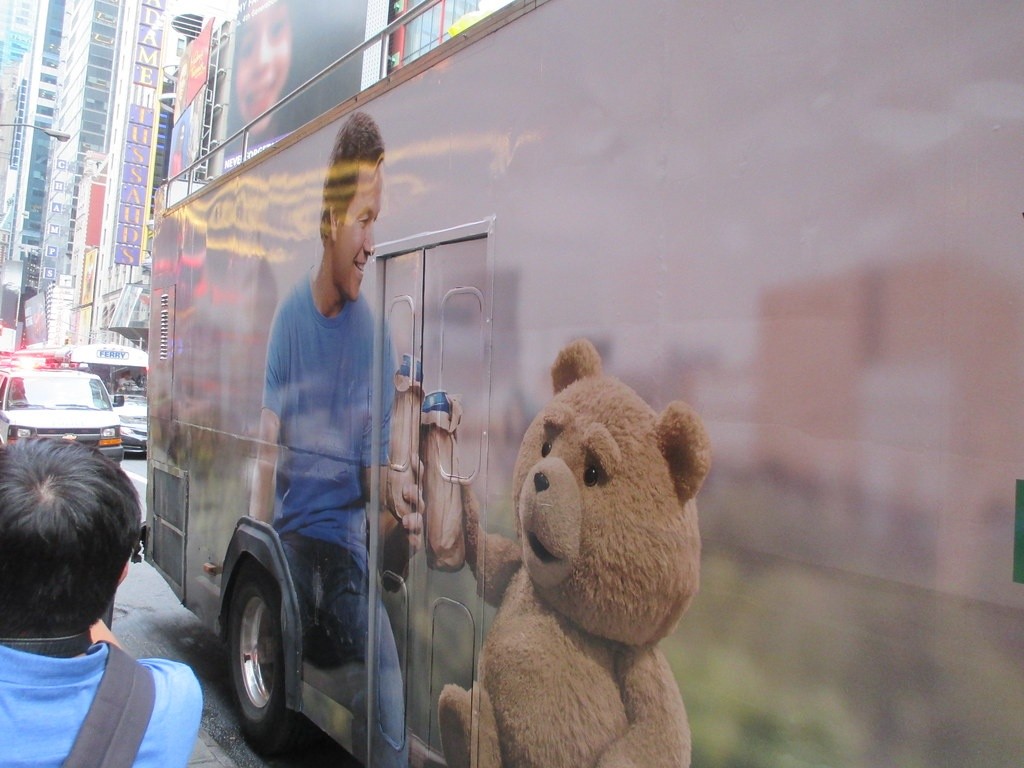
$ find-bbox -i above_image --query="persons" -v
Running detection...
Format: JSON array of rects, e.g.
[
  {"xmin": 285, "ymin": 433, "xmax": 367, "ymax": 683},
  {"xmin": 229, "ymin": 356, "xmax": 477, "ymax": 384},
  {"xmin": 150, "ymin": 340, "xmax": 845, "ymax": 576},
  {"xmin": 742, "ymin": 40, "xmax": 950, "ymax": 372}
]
[
  {"xmin": 118, "ymin": 369, "xmax": 138, "ymax": 390},
  {"xmin": 168, "ymin": 95, "xmax": 198, "ymax": 178},
  {"xmin": 233, "ymin": 0, "xmax": 298, "ymax": 152},
  {"xmin": 0, "ymin": 437, "xmax": 203, "ymax": 768},
  {"xmin": 249, "ymin": 112, "xmax": 423, "ymax": 768}
]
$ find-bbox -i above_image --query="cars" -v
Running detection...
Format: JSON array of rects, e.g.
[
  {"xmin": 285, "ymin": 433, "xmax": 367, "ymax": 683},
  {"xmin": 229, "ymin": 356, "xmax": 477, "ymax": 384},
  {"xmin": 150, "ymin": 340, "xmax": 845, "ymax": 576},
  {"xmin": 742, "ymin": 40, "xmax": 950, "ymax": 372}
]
[{"xmin": 108, "ymin": 393, "xmax": 147, "ymax": 453}]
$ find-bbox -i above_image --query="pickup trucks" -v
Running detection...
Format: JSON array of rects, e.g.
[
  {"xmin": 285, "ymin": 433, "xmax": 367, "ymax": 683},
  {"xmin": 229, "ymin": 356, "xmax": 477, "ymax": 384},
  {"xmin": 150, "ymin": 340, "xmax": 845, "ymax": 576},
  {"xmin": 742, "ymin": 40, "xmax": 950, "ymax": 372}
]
[{"xmin": 0, "ymin": 357, "xmax": 124, "ymax": 466}]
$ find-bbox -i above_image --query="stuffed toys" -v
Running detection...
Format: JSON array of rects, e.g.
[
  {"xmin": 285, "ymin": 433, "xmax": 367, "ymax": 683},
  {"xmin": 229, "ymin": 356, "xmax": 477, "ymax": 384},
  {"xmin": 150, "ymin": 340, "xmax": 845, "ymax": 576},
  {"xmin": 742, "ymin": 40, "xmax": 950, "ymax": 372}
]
[{"xmin": 438, "ymin": 338, "xmax": 711, "ymax": 768}]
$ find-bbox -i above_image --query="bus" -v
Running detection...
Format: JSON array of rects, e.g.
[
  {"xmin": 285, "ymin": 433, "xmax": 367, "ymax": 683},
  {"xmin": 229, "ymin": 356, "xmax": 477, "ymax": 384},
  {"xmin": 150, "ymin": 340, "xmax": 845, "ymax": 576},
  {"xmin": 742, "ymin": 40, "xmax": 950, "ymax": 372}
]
[
  {"xmin": 147, "ymin": 0, "xmax": 1024, "ymax": 768},
  {"xmin": 8, "ymin": 343, "xmax": 148, "ymax": 394}
]
